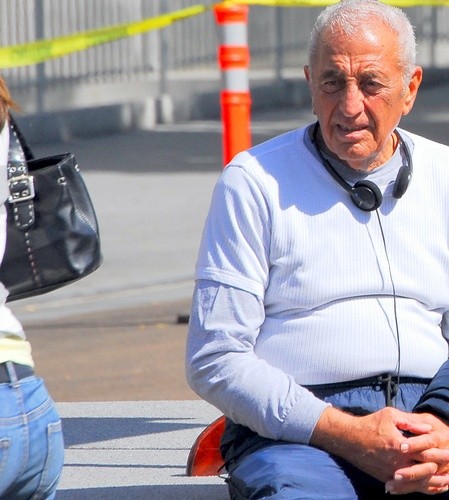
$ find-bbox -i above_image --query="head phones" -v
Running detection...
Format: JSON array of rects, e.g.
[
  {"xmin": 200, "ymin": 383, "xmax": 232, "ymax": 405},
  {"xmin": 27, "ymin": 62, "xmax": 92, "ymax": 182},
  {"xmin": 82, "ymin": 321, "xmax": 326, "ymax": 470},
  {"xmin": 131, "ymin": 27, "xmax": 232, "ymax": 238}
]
[{"xmin": 311, "ymin": 119, "xmax": 413, "ymax": 212}]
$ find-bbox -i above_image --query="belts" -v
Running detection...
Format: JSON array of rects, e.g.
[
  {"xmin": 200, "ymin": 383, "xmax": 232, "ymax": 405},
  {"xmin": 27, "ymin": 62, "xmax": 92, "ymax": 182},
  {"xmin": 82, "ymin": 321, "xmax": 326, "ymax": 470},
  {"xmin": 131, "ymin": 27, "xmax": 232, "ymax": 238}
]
[{"xmin": 0, "ymin": 361, "xmax": 38, "ymax": 386}]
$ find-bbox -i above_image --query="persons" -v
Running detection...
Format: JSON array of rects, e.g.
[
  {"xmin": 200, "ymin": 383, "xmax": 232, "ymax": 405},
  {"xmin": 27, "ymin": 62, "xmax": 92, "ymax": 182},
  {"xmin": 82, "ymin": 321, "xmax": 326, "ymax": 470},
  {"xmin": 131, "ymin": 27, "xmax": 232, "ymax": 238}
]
[
  {"xmin": 184, "ymin": 0, "xmax": 449, "ymax": 500},
  {"xmin": 0, "ymin": 73, "xmax": 64, "ymax": 500}
]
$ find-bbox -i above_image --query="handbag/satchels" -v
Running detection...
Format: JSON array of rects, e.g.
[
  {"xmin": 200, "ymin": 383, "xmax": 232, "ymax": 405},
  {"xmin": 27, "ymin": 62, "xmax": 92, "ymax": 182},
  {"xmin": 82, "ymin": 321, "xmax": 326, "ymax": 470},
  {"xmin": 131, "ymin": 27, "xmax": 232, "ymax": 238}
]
[{"xmin": 0, "ymin": 109, "xmax": 104, "ymax": 303}]
